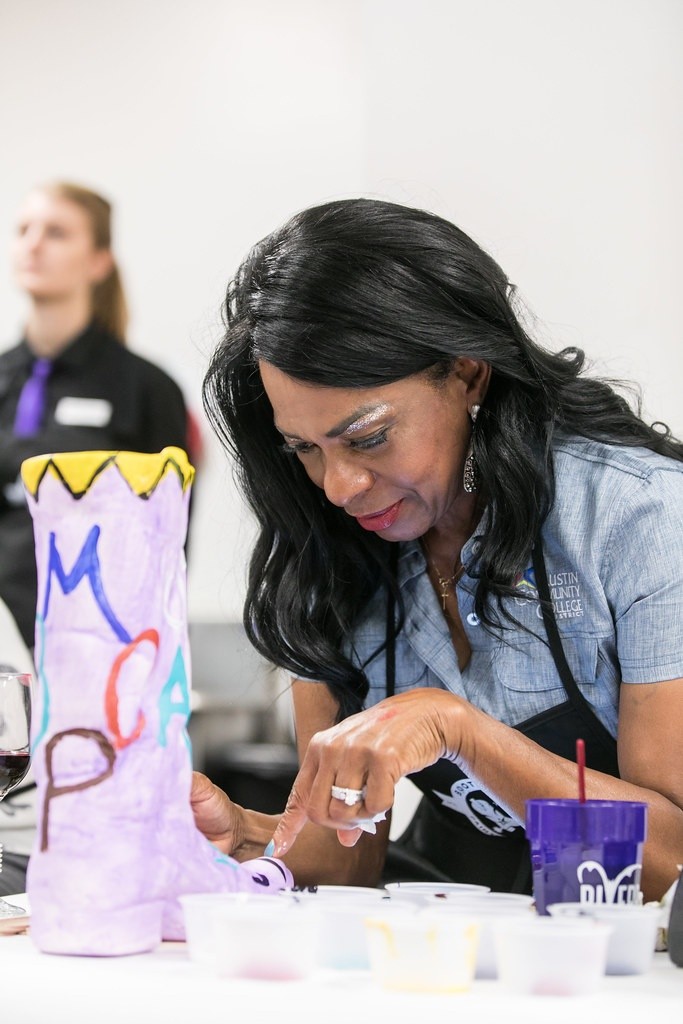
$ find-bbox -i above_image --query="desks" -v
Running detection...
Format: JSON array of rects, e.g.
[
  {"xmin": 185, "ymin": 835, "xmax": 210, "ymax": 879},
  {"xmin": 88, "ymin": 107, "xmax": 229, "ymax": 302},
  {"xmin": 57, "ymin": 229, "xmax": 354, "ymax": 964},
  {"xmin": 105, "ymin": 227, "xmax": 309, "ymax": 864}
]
[{"xmin": 0, "ymin": 892, "xmax": 683, "ymax": 1024}]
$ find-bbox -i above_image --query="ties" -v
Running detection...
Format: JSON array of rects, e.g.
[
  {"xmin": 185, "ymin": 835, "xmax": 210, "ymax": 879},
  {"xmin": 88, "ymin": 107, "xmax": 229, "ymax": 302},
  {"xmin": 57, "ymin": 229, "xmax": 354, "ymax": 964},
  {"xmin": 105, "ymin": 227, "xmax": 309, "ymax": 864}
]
[{"xmin": 15, "ymin": 355, "xmax": 52, "ymax": 441}]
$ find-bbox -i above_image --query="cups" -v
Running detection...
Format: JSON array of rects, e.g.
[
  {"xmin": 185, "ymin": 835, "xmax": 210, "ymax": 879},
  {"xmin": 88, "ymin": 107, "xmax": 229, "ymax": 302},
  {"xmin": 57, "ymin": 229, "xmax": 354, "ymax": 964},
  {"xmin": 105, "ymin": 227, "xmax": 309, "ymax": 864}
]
[{"xmin": 528, "ymin": 799, "xmax": 648, "ymax": 916}]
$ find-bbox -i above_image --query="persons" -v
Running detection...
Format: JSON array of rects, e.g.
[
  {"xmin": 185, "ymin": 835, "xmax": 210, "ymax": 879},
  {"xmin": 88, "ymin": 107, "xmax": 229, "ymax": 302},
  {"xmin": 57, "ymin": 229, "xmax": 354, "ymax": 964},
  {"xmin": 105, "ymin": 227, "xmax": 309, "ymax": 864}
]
[
  {"xmin": 191, "ymin": 197, "xmax": 683, "ymax": 905},
  {"xmin": 0, "ymin": 183, "xmax": 188, "ymax": 649}
]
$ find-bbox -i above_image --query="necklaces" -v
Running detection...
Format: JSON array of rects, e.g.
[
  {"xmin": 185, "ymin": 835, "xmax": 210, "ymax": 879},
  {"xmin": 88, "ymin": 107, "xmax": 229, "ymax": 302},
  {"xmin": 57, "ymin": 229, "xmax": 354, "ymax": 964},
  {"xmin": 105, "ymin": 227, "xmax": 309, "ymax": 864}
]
[{"xmin": 422, "ymin": 535, "xmax": 464, "ymax": 611}]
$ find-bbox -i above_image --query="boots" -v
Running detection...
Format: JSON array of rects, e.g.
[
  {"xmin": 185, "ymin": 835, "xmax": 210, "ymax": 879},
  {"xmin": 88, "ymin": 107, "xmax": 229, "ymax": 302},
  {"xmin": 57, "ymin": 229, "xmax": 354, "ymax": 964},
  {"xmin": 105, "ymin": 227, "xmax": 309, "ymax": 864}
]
[{"xmin": 21, "ymin": 448, "xmax": 293, "ymax": 957}]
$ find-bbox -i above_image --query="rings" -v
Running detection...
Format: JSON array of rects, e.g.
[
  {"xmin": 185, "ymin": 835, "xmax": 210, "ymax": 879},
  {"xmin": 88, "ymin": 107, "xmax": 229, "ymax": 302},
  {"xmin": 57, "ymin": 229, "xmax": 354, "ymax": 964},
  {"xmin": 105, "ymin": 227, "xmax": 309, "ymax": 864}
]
[{"xmin": 331, "ymin": 785, "xmax": 364, "ymax": 806}]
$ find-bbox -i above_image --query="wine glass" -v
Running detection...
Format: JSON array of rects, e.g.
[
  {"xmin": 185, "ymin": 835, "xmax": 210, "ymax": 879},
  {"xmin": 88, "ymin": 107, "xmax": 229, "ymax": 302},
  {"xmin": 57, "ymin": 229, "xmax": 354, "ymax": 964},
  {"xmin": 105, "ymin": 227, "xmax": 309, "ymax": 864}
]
[{"xmin": 0, "ymin": 672, "xmax": 33, "ymax": 914}]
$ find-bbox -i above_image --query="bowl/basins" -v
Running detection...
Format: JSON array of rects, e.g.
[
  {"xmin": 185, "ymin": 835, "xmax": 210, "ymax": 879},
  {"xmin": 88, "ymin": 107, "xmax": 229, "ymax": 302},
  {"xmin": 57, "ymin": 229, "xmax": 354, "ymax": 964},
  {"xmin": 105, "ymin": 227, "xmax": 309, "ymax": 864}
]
[{"xmin": 179, "ymin": 880, "xmax": 658, "ymax": 995}]
